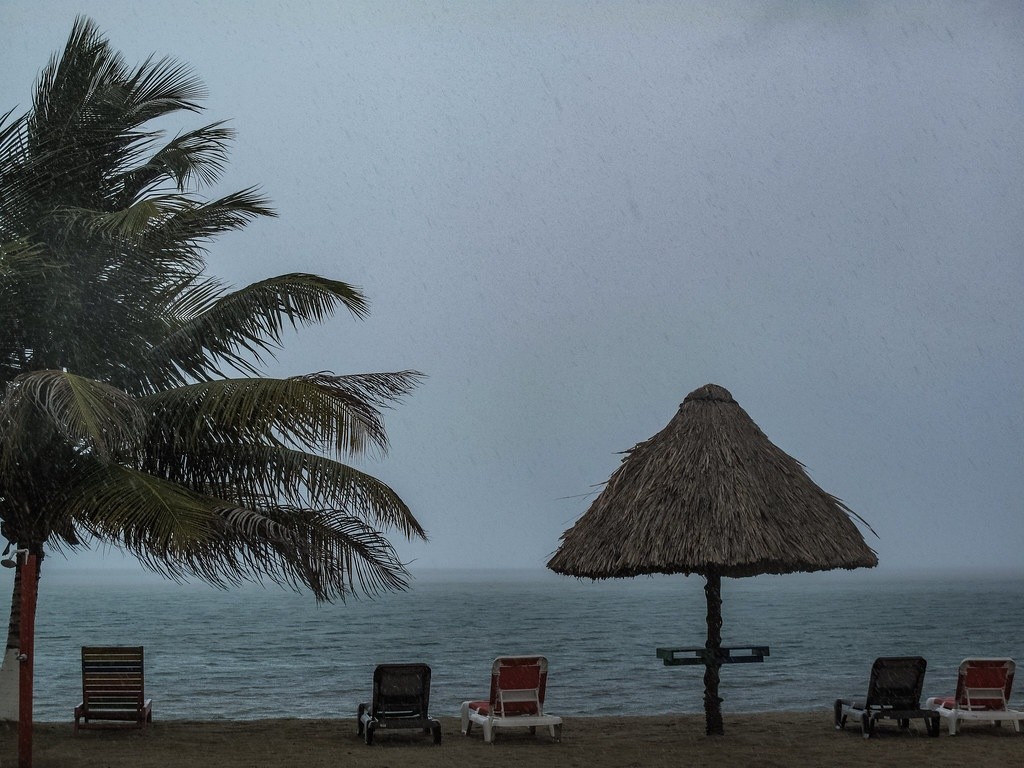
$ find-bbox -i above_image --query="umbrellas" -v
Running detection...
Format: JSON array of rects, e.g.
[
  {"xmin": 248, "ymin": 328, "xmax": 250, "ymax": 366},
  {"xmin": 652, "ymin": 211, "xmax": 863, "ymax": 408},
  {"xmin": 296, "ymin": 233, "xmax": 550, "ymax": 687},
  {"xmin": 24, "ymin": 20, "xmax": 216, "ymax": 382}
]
[{"xmin": 546, "ymin": 384, "xmax": 879, "ymax": 734}]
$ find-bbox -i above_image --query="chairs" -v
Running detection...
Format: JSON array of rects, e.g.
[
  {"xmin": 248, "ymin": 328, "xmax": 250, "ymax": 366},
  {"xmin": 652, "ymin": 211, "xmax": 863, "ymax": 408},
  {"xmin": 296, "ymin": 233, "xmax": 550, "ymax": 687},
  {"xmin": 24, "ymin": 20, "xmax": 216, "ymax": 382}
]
[
  {"xmin": 833, "ymin": 656, "xmax": 941, "ymax": 741},
  {"xmin": 356, "ymin": 663, "xmax": 442, "ymax": 748},
  {"xmin": 458, "ymin": 654, "xmax": 563, "ymax": 746},
  {"xmin": 926, "ymin": 656, "xmax": 1024, "ymax": 737},
  {"xmin": 72, "ymin": 645, "xmax": 153, "ymax": 738}
]
